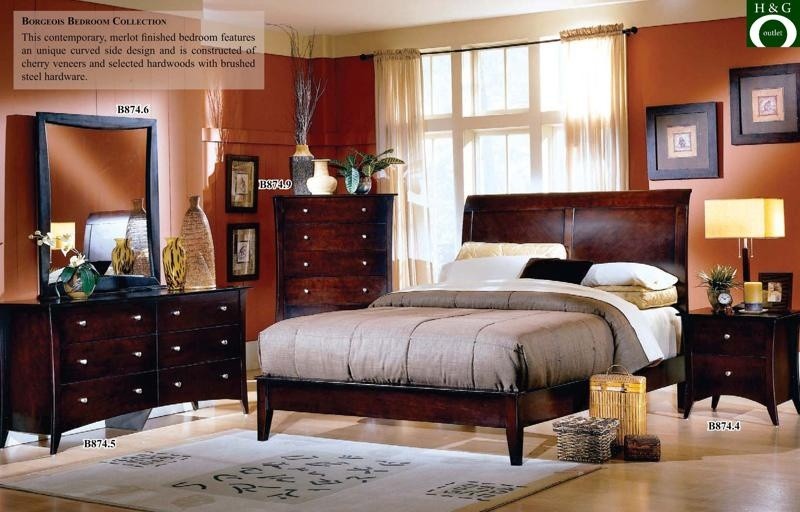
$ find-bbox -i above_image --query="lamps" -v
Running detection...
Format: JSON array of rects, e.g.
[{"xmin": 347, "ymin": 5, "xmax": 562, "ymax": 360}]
[{"xmin": 703, "ymin": 197, "xmax": 786, "ymax": 282}]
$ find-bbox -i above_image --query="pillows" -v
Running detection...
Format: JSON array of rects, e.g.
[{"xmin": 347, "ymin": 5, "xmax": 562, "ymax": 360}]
[
  {"xmin": 435, "ymin": 257, "xmax": 528, "ymax": 280},
  {"xmin": 581, "ymin": 261, "xmax": 679, "ymax": 291}
]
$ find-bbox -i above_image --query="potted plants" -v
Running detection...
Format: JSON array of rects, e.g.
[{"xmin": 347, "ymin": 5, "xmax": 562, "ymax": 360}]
[
  {"xmin": 331, "ymin": 148, "xmax": 404, "ymax": 194},
  {"xmin": 264, "ymin": 23, "xmax": 326, "ymax": 194},
  {"xmin": 699, "ymin": 264, "xmax": 738, "ymax": 309}
]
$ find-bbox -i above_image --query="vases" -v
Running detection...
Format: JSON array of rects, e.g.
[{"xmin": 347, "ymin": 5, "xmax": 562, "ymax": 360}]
[
  {"xmin": 307, "ymin": 159, "xmax": 337, "ymax": 196},
  {"xmin": 63, "ymin": 269, "xmax": 96, "ymax": 301}
]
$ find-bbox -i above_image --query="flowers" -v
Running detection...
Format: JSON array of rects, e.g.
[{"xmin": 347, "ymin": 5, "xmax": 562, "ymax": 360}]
[{"xmin": 27, "ymin": 232, "xmax": 101, "ymax": 294}]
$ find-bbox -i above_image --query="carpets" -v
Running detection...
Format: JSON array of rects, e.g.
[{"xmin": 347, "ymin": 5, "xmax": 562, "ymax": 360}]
[{"xmin": 0, "ymin": 429, "xmax": 603, "ymax": 510}]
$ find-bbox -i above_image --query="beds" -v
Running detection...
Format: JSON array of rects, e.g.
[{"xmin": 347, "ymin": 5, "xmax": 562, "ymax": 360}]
[{"xmin": 255, "ymin": 188, "xmax": 691, "ymax": 468}]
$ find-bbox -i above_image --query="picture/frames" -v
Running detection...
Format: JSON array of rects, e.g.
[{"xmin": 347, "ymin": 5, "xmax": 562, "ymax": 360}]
[
  {"xmin": 224, "ymin": 152, "xmax": 259, "ymax": 214},
  {"xmin": 226, "ymin": 222, "xmax": 258, "ymax": 282},
  {"xmin": 726, "ymin": 62, "xmax": 799, "ymax": 148},
  {"xmin": 644, "ymin": 101, "xmax": 721, "ymax": 182}
]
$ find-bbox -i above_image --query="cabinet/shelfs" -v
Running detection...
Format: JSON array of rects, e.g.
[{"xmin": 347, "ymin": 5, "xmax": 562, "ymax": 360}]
[
  {"xmin": 0, "ymin": 285, "xmax": 253, "ymax": 455},
  {"xmin": 273, "ymin": 193, "xmax": 395, "ymax": 322}
]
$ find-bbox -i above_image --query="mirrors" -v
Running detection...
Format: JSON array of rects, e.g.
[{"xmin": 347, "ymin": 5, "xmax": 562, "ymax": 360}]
[{"xmin": 35, "ymin": 111, "xmax": 161, "ymax": 293}]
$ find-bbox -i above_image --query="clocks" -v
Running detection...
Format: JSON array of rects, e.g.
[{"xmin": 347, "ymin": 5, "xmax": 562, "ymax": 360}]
[
  {"xmin": 757, "ymin": 271, "xmax": 793, "ymax": 311},
  {"xmin": 718, "ymin": 292, "xmax": 733, "ymax": 311}
]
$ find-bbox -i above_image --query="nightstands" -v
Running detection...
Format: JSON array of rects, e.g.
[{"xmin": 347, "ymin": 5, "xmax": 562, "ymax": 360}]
[{"xmin": 681, "ymin": 304, "xmax": 799, "ymax": 427}]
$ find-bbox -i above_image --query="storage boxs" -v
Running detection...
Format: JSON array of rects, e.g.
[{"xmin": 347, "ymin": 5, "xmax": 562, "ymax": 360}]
[{"xmin": 589, "ymin": 373, "xmax": 647, "ymax": 446}]
